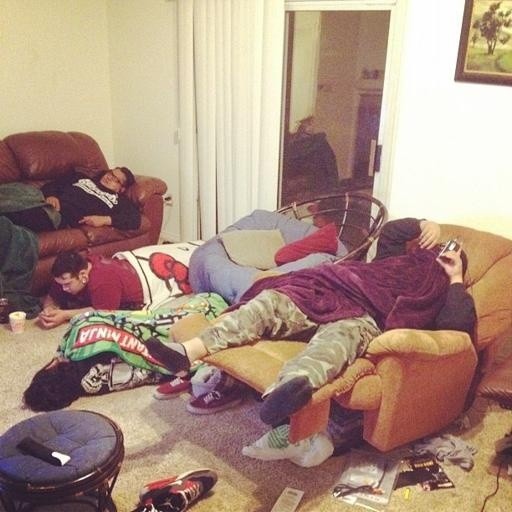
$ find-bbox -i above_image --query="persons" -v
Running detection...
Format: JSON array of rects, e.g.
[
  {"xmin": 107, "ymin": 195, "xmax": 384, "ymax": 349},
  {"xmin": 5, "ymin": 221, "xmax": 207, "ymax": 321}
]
[
  {"xmin": 129, "ymin": 468, "xmax": 217, "ymax": 511},
  {"xmin": 36, "ymin": 240, "xmax": 205, "ymax": 329},
  {"xmin": 22, "ymin": 292, "xmax": 230, "ymax": 412},
  {"xmin": 6, "ymin": 167, "xmax": 141, "ymax": 232},
  {"xmin": 144, "ymin": 216, "xmax": 476, "ymax": 426}
]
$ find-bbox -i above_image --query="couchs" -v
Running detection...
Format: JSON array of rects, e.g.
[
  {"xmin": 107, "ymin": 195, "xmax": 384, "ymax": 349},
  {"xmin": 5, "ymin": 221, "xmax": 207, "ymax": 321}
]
[
  {"xmin": 0, "ymin": 126, "xmax": 168, "ymax": 303},
  {"xmin": 283, "ymin": 141, "xmax": 334, "ymax": 209}
]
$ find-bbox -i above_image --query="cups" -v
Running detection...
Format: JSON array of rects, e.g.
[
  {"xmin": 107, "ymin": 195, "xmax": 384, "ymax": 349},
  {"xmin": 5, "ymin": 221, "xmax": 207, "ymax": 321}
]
[{"xmin": 10, "ymin": 311, "xmax": 27, "ymax": 333}]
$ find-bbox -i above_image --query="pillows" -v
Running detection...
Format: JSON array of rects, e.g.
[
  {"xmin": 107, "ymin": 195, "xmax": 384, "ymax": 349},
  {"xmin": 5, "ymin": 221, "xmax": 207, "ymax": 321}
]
[
  {"xmin": 216, "ymin": 228, "xmax": 287, "ymax": 270},
  {"xmin": 276, "ymin": 220, "xmax": 340, "ymax": 265}
]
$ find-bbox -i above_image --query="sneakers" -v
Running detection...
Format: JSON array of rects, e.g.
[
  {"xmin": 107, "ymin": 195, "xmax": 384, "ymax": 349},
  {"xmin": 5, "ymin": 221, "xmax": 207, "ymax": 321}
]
[
  {"xmin": 241, "ymin": 424, "xmax": 309, "ymax": 462},
  {"xmin": 186, "ymin": 381, "xmax": 243, "ymax": 416},
  {"xmin": 153, "ymin": 376, "xmax": 192, "ymax": 400},
  {"xmin": 139, "ymin": 467, "xmax": 219, "ymax": 512}
]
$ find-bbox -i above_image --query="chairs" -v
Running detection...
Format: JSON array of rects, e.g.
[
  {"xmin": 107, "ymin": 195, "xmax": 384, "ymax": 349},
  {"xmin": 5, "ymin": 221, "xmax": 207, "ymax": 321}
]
[
  {"xmin": 169, "ymin": 219, "xmax": 512, "ymax": 454},
  {"xmin": 187, "ymin": 189, "xmax": 390, "ymax": 306}
]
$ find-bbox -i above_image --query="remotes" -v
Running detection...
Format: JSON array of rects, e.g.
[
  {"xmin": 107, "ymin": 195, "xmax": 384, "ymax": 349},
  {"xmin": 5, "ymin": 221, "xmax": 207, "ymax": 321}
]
[{"xmin": 17, "ymin": 436, "xmax": 72, "ymax": 468}]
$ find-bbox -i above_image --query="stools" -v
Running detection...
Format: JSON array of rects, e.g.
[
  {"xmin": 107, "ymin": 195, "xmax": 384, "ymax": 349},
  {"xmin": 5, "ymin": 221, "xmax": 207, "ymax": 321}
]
[{"xmin": 1, "ymin": 408, "xmax": 127, "ymax": 512}]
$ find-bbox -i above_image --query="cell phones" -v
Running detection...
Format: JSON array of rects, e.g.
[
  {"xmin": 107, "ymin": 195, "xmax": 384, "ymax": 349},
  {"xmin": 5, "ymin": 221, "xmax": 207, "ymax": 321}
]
[{"xmin": 439, "ymin": 240, "xmax": 461, "ymax": 264}]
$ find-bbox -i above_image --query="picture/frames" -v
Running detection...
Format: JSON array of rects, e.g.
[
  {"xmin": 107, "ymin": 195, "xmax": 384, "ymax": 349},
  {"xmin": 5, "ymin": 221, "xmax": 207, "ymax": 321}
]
[{"xmin": 452, "ymin": 0, "xmax": 512, "ymax": 85}]
